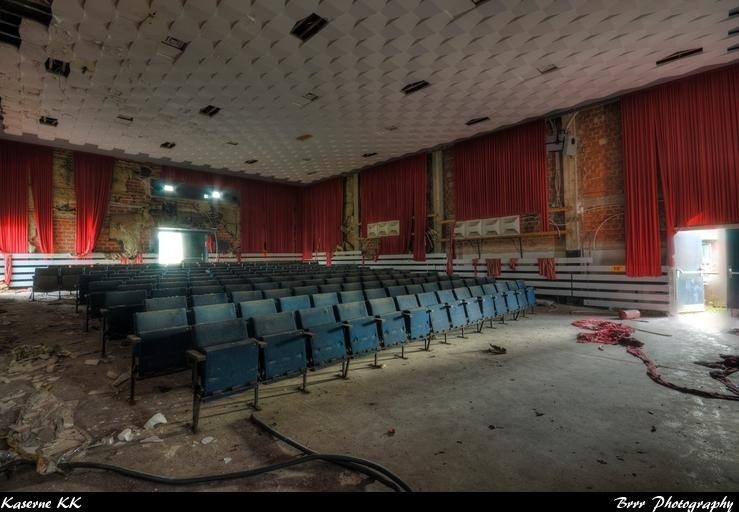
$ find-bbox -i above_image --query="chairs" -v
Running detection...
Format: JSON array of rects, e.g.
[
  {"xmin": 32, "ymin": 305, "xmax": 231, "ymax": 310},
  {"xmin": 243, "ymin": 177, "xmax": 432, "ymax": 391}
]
[{"xmin": 29, "ymin": 263, "xmax": 538, "ymax": 433}]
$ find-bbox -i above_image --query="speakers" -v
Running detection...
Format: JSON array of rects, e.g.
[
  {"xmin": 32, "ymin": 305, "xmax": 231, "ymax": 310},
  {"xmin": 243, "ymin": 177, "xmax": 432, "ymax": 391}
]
[{"xmin": 563, "ymin": 135, "xmax": 577, "ymax": 156}]
